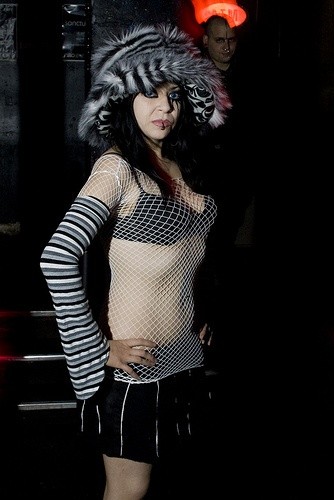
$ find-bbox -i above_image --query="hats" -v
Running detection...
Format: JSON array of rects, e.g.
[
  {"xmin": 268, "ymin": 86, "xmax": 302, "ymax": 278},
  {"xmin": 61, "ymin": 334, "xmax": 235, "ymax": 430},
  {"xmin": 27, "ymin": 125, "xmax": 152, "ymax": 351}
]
[{"xmin": 78, "ymin": 23, "xmax": 232, "ymax": 152}]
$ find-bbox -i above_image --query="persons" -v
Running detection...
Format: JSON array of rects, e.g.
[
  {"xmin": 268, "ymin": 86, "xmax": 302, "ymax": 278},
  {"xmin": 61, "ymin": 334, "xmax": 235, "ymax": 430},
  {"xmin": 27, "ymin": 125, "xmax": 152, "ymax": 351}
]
[
  {"xmin": 187, "ymin": 15, "xmax": 263, "ymax": 375},
  {"xmin": 38, "ymin": 25, "xmax": 224, "ymax": 500}
]
